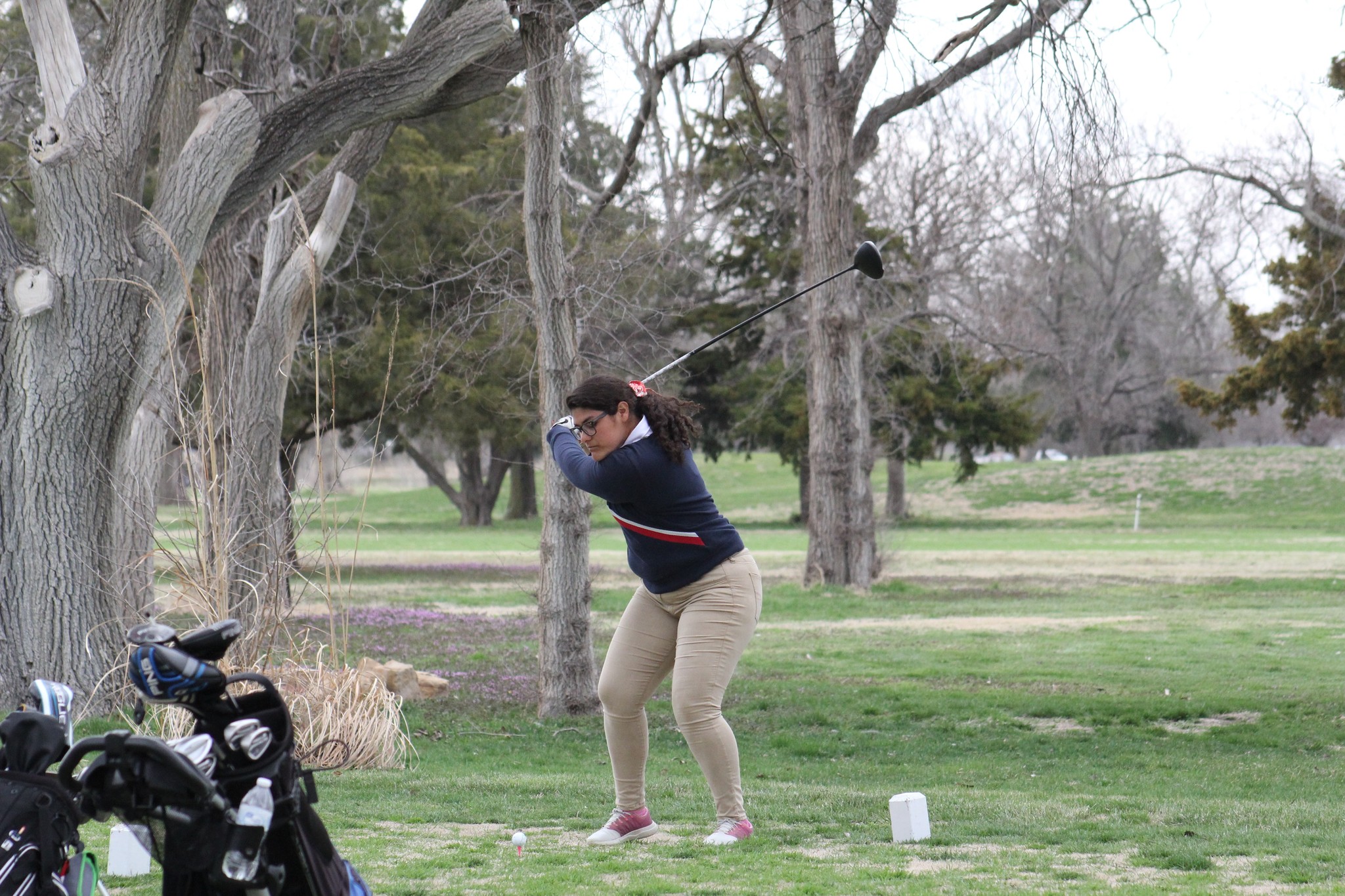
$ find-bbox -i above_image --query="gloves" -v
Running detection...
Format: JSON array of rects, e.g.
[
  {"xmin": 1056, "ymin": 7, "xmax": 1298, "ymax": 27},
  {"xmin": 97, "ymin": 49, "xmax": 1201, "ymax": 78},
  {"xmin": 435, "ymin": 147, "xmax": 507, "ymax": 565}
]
[{"xmin": 550, "ymin": 416, "xmax": 580, "ymax": 434}]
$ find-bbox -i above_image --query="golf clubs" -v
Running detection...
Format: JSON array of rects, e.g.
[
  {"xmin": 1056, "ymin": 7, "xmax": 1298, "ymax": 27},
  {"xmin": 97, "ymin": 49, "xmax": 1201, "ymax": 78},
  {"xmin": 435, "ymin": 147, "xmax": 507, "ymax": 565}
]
[
  {"xmin": 638, "ymin": 241, "xmax": 886, "ymax": 382},
  {"xmin": 16, "ymin": 618, "xmax": 277, "ymax": 790}
]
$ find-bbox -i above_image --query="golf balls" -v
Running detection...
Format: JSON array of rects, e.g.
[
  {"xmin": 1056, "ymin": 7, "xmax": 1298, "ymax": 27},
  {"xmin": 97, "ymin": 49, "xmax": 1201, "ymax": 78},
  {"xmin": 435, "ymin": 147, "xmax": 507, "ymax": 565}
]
[{"xmin": 512, "ymin": 832, "xmax": 527, "ymax": 847}]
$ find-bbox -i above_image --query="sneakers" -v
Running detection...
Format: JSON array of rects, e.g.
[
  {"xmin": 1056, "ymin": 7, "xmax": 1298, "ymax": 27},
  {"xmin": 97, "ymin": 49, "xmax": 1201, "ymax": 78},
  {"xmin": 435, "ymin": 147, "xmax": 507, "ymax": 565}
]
[
  {"xmin": 703, "ymin": 819, "xmax": 753, "ymax": 845},
  {"xmin": 586, "ymin": 807, "xmax": 658, "ymax": 846}
]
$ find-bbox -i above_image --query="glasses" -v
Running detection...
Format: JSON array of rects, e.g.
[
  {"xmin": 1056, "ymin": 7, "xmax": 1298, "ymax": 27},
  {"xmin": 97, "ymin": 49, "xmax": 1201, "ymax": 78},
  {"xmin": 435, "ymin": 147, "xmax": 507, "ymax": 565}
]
[{"xmin": 569, "ymin": 411, "xmax": 608, "ymax": 440}]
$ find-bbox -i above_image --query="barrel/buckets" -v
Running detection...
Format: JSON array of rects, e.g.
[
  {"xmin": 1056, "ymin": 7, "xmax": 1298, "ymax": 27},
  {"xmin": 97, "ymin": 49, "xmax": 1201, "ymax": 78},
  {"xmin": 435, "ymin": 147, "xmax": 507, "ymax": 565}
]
[{"xmin": 107, "ymin": 823, "xmax": 151, "ymax": 876}]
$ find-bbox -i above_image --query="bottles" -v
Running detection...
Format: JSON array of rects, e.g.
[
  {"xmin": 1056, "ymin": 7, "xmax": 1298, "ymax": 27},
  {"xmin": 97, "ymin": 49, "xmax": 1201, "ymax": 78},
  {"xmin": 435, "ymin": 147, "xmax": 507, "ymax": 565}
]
[{"xmin": 220, "ymin": 777, "xmax": 274, "ymax": 881}]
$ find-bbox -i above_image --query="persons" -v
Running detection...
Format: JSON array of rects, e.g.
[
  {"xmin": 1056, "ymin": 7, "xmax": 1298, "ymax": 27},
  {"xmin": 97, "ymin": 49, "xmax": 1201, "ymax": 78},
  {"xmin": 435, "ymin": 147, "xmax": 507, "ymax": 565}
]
[{"xmin": 546, "ymin": 375, "xmax": 763, "ymax": 846}]
